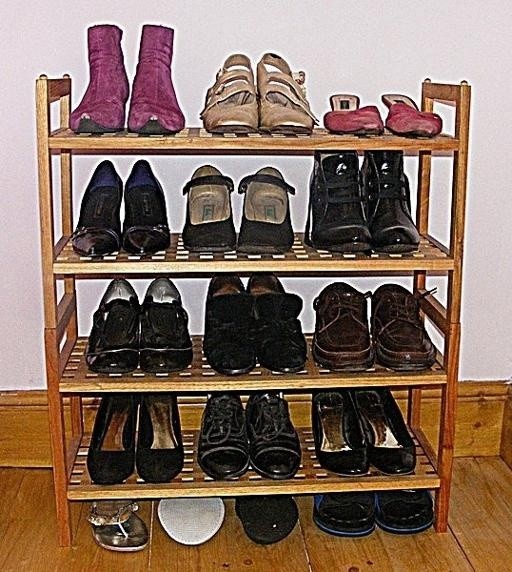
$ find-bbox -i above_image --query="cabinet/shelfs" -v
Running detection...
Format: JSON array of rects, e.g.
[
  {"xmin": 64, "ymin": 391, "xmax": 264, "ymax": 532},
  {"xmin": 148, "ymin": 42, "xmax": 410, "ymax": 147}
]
[{"xmin": 36, "ymin": 73, "xmax": 472, "ymax": 549}]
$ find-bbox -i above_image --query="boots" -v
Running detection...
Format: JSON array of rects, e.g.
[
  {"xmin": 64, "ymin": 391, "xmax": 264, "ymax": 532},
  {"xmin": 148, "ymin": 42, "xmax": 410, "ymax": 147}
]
[
  {"xmin": 128, "ymin": 25, "xmax": 185, "ymax": 135},
  {"xmin": 69, "ymin": 25, "xmax": 129, "ymax": 133}
]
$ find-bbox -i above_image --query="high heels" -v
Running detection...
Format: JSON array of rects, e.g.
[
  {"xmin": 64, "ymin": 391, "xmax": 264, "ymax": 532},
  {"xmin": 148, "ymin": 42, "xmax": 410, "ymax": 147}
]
[
  {"xmin": 381, "ymin": 94, "xmax": 442, "ymax": 137},
  {"xmin": 323, "ymin": 94, "xmax": 384, "ymax": 135}
]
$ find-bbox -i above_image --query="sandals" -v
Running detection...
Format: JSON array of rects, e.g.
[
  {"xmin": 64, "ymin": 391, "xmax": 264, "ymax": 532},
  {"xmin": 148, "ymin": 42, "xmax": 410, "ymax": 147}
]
[
  {"xmin": 199, "ymin": 54, "xmax": 260, "ymax": 133},
  {"xmin": 257, "ymin": 53, "xmax": 319, "ymax": 133}
]
